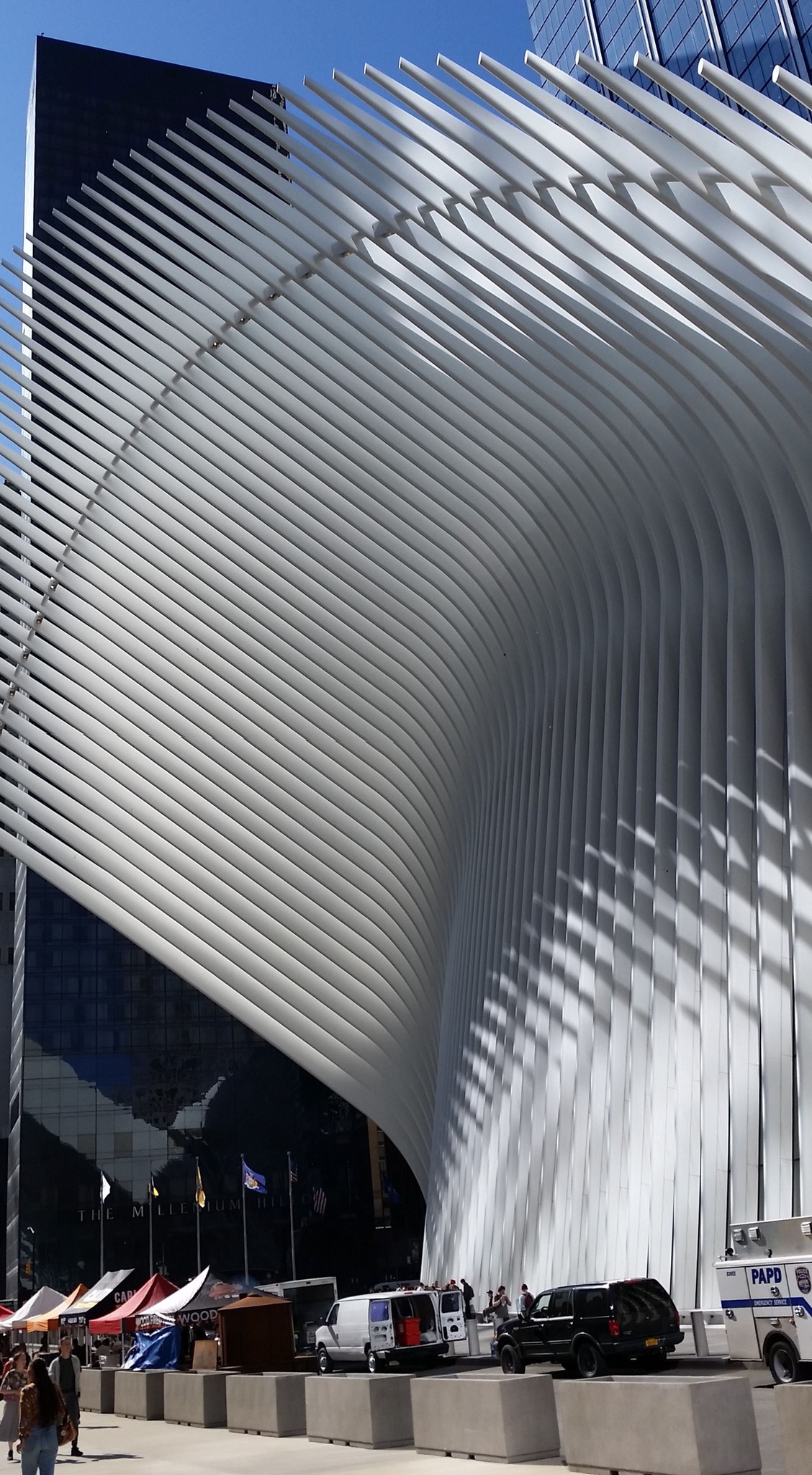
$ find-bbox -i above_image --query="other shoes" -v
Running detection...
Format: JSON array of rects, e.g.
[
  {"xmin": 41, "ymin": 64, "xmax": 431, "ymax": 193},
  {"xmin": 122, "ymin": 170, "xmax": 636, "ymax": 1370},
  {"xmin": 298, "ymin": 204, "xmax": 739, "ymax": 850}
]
[
  {"xmin": 7, "ymin": 1450, "xmax": 13, "ymax": 1460},
  {"xmin": 71, "ymin": 1448, "xmax": 82, "ymax": 1456}
]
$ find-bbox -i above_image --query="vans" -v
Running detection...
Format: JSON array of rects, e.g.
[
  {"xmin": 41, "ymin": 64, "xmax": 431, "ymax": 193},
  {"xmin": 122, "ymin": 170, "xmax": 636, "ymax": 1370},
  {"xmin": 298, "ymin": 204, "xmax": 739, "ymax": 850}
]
[{"xmin": 312, "ymin": 1289, "xmax": 467, "ymax": 1374}]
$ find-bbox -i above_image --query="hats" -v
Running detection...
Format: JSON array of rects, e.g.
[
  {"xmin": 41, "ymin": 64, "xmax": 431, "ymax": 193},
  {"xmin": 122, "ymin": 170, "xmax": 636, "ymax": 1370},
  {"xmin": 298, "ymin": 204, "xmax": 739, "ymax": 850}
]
[
  {"xmin": 497, "ymin": 1285, "xmax": 506, "ymax": 1292},
  {"xmin": 451, "ymin": 1280, "xmax": 455, "ymax": 1284},
  {"xmin": 520, "ymin": 1283, "xmax": 528, "ymax": 1290},
  {"xmin": 487, "ymin": 1290, "xmax": 493, "ymax": 1294}
]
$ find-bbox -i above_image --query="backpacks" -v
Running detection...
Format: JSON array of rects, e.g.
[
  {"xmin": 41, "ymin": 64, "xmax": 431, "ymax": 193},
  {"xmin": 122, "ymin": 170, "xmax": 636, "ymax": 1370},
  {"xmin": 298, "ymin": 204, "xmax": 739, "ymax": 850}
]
[
  {"xmin": 465, "ymin": 1285, "xmax": 474, "ymax": 1297},
  {"xmin": 492, "ymin": 1294, "xmax": 508, "ymax": 1311},
  {"xmin": 522, "ymin": 1292, "xmax": 537, "ymax": 1311}
]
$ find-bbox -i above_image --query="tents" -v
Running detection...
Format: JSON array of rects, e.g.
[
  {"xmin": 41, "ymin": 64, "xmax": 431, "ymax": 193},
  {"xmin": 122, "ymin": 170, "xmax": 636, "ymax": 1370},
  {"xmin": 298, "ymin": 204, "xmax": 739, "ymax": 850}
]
[{"xmin": 0, "ymin": 1269, "xmax": 295, "ymax": 1367}]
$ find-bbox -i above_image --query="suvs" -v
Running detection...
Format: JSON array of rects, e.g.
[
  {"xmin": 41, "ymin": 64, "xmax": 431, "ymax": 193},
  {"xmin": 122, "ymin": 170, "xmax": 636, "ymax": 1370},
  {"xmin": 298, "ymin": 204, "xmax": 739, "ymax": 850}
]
[{"xmin": 491, "ymin": 1276, "xmax": 685, "ymax": 1378}]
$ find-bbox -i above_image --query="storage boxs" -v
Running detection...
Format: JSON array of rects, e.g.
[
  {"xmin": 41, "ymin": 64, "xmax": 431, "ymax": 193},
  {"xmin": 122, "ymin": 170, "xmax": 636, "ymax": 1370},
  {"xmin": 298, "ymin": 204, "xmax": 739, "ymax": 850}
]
[{"xmin": 395, "ymin": 1317, "xmax": 421, "ymax": 1346}]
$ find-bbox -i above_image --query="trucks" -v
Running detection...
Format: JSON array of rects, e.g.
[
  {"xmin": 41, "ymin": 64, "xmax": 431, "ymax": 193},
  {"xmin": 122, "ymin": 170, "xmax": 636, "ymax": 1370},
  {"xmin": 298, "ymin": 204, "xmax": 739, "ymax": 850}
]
[
  {"xmin": 713, "ymin": 1215, "xmax": 811, "ymax": 1384},
  {"xmin": 253, "ymin": 1275, "xmax": 339, "ymax": 1357}
]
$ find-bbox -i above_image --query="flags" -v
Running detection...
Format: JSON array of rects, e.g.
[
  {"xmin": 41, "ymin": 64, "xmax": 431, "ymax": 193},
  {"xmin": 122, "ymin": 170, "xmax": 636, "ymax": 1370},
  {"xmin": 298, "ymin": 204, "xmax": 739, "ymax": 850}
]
[
  {"xmin": 100, "ymin": 1173, "xmax": 111, "ymax": 1203},
  {"xmin": 289, "ymin": 1158, "xmax": 326, "ymax": 1214},
  {"xmin": 195, "ymin": 1164, "xmax": 206, "ymax": 1208},
  {"xmin": 243, "ymin": 1159, "xmax": 268, "ymax": 1194},
  {"xmin": 148, "ymin": 1171, "xmax": 159, "ymax": 1197}
]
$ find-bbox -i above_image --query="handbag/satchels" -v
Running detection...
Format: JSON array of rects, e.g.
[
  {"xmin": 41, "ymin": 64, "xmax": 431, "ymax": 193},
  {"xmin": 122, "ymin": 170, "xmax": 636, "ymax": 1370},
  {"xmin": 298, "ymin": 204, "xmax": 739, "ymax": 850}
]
[{"xmin": 57, "ymin": 1421, "xmax": 77, "ymax": 1447}]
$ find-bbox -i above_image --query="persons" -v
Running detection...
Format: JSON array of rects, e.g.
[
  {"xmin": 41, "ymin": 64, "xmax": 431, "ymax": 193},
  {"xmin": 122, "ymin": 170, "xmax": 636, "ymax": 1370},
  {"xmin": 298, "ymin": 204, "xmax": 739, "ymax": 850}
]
[
  {"xmin": 102, "ymin": 1337, "xmax": 111, "ymax": 1348},
  {"xmin": 93, "ymin": 1339, "xmax": 110, "ymax": 1360},
  {"xmin": 461, "ymin": 1279, "xmax": 472, "ymax": 1319},
  {"xmin": 519, "ymin": 1283, "xmax": 536, "ymax": 1311},
  {"xmin": 481, "ymin": 1289, "xmax": 495, "ymax": 1323},
  {"xmin": 48, "ymin": 1335, "xmax": 84, "ymax": 1456},
  {"xmin": 16, "ymin": 1356, "xmax": 66, "ymax": 1475},
  {"xmin": 39, "ymin": 1332, "xmax": 51, "ymax": 1353},
  {"xmin": 492, "ymin": 1285, "xmax": 512, "ymax": 1322},
  {"xmin": 70, "ymin": 1337, "xmax": 87, "ymax": 1364},
  {"xmin": 0, "ymin": 1345, "xmax": 31, "ymax": 1459},
  {"xmin": 374, "ymin": 1279, "xmax": 460, "ymax": 1292}
]
